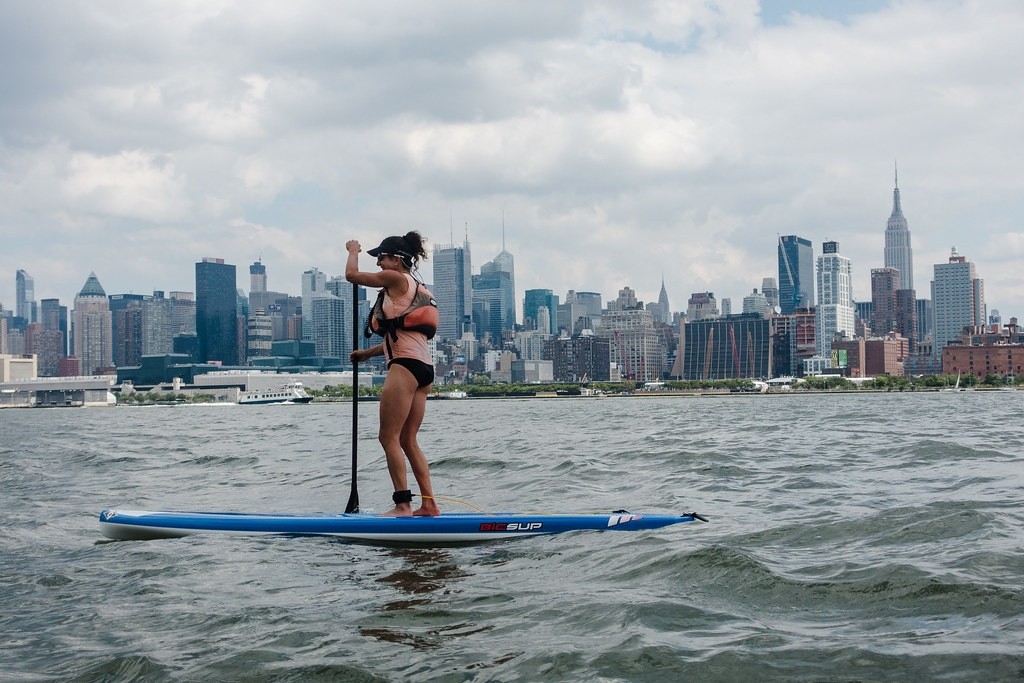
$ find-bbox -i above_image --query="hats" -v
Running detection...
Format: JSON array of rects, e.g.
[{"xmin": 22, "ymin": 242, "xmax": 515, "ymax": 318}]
[{"xmin": 366, "ymin": 239, "xmax": 414, "ymax": 258}]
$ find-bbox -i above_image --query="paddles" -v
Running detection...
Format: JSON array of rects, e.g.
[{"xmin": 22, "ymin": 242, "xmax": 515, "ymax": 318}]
[{"xmin": 343, "ymin": 282, "xmax": 360, "ymax": 515}]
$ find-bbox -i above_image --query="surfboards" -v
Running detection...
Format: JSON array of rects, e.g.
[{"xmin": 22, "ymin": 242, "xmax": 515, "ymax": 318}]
[{"xmin": 100, "ymin": 508, "xmax": 693, "ymax": 544}]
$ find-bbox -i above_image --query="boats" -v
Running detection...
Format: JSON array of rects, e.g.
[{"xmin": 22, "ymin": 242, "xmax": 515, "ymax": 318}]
[
  {"xmin": 237, "ymin": 381, "xmax": 314, "ymax": 405},
  {"xmin": 98, "ymin": 509, "xmax": 709, "ymax": 543}
]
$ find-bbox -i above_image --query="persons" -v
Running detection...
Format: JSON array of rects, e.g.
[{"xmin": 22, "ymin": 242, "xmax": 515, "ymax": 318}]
[{"xmin": 346, "ymin": 231, "xmax": 441, "ymax": 518}]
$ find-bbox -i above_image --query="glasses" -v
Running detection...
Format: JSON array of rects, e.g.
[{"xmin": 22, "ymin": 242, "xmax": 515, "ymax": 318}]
[{"xmin": 377, "ymin": 251, "xmax": 405, "ymax": 259}]
